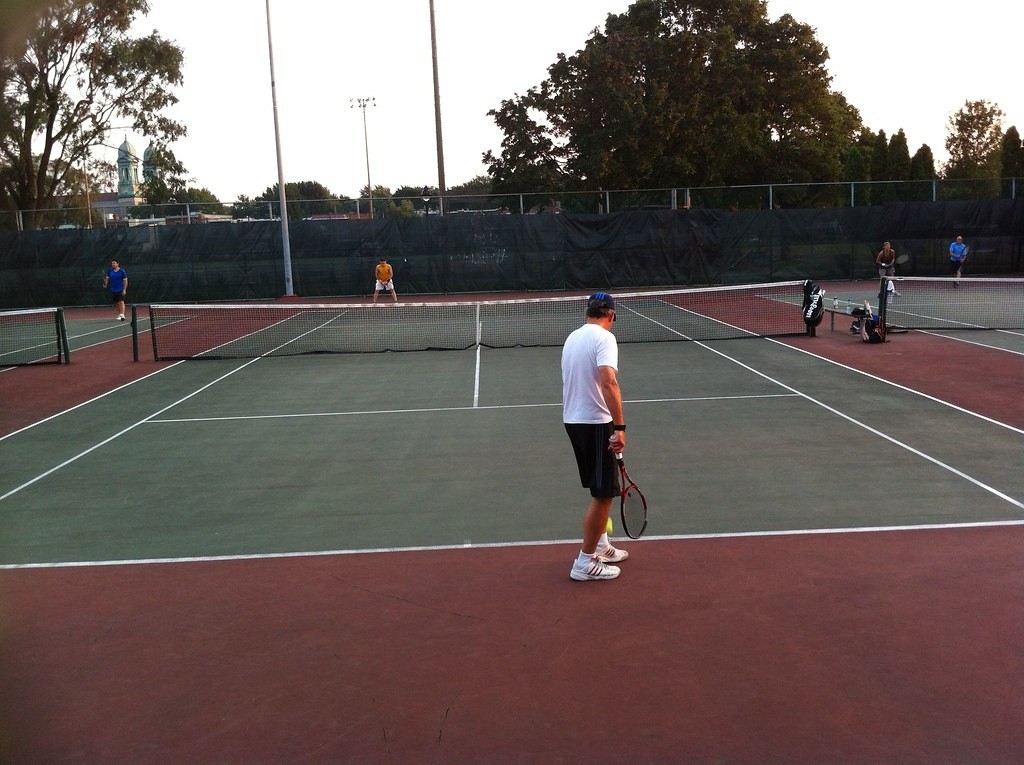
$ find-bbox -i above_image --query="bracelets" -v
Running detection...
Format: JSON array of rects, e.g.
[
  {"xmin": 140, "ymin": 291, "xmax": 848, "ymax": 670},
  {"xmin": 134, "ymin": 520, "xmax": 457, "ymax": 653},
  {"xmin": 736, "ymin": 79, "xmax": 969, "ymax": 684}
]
[
  {"xmin": 882, "ymin": 264, "xmax": 886, "ymax": 267},
  {"xmin": 614, "ymin": 424, "xmax": 626, "ymax": 432}
]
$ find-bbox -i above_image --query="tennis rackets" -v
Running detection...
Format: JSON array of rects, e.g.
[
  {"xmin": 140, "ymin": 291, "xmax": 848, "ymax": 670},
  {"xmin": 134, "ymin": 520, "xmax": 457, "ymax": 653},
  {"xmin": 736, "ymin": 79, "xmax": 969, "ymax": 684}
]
[
  {"xmin": 103, "ymin": 270, "xmax": 110, "ymax": 286},
  {"xmin": 610, "ymin": 434, "xmax": 648, "ymax": 539},
  {"xmin": 961, "ymin": 245, "xmax": 971, "ymax": 257},
  {"xmin": 889, "ymin": 254, "xmax": 909, "ymax": 267}
]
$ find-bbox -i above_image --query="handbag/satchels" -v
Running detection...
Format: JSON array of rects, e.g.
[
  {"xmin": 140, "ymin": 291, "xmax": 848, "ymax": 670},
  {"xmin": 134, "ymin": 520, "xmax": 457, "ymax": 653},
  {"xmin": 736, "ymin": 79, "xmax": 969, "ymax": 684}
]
[{"xmin": 850, "ymin": 307, "xmax": 866, "ymax": 317}]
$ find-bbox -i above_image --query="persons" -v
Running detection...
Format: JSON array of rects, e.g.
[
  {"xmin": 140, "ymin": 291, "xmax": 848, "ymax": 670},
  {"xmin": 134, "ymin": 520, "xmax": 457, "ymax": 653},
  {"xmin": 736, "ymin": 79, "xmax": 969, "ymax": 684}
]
[
  {"xmin": 876, "ymin": 241, "xmax": 902, "ymax": 299},
  {"xmin": 103, "ymin": 259, "xmax": 128, "ymax": 321},
  {"xmin": 373, "ymin": 258, "xmax": 398, "ymax": 303},
  {"xmin": 949, "ymin": 236, "xmax": 967, "ymax": 289},
  {"xmin": 560, "ymin": 292, "xmax": 630, "ymax": 581}
]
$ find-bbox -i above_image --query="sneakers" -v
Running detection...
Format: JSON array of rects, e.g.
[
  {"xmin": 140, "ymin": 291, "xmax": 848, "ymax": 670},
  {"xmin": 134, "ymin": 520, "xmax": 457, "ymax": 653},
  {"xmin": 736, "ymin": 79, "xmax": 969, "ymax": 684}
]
[
  {"xmin": 570, "ymin": 556, "xmax": 620, "ymax": 580},
  {"xmin": 597, "ymin": 543, "xmax": 628, "ymax": 562}
]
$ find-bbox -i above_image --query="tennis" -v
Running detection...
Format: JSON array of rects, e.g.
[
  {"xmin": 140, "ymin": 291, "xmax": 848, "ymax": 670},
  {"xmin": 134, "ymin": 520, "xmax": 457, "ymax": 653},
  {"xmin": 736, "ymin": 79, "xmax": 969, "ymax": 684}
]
[{"xmin": 605, "ymin": 516, "xmax": 613, "ymax": 534}]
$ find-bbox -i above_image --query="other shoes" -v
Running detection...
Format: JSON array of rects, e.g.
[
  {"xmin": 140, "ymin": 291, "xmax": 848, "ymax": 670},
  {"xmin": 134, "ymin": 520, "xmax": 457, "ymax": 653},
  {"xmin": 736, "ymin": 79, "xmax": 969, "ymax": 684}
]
[
  {"xmin": 953, "ymin": 281, "xmax": 959, "ymax": 288},
  {"xmin": 116, "ymin": 315, "xmax": 125, "ymax": 321},
  {"xmin": 894, "ymin": 292, "xmax": 902, "ymax": 297}
]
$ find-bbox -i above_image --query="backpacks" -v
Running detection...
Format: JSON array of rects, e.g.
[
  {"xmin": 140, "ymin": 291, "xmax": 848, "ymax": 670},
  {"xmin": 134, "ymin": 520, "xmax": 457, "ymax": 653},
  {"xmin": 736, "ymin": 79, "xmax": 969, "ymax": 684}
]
[{"xmin": 859, "ymin": 314, "xmax": 885, "ymax": 343}]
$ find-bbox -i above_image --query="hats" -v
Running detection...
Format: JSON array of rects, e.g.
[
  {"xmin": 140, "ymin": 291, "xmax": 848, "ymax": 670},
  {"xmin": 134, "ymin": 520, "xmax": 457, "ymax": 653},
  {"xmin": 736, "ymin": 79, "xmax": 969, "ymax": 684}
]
[
  {"xmin": 381, "ymin": 258, "xmax": 385, "ymax": 261},
  {"xmin": 588, "ymin": 292, "xmax": 616, "ymax": 322}
]
